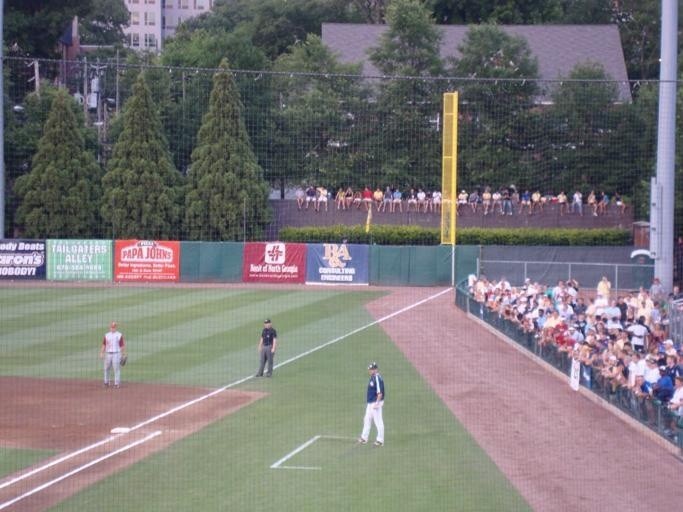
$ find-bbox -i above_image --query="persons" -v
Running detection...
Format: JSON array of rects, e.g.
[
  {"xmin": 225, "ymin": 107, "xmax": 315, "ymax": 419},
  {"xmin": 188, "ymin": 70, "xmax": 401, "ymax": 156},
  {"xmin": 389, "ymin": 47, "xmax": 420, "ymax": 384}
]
[
  {"xmin": 255, "ymin": 319, "xmax": 277, "ymax": 377},
  {"xmin": 541, "ymin": 189, "xmax": 583, "ymax": 216},
  {"xmin": 456, "ymin": 188, "xmax": 543, "ymax": 216},
  {"xmin": 466, "ymin": 273, "xmax": 683, "ymax": 443},
  {"xmin": 587, "ymin": 189, "xmax": 624, "ymax": 216},
  {"xmin": 358, "ymin": 362, "xmax": 384, "ymax": 447},
  {"xmin": 297, "ymin": 186, "xmax": 328, "ymax": 212},
  {"xmin": 334, "ymin": 186, "xmax": 403, "ymax": 213},
  {"xmin": 407, "ymin": 188, "xmax": 442, "ymax": 213},
  {"xmin": 100, "ymin": 322, "xmax": 126, "ymax": 388}
]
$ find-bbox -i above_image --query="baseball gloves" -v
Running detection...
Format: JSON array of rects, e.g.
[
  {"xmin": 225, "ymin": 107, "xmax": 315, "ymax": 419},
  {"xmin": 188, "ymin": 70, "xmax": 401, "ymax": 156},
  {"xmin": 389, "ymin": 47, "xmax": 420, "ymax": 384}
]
[{"xmin": 119, "ymin": 354, "xmax": 127, "ymax": 365}]
